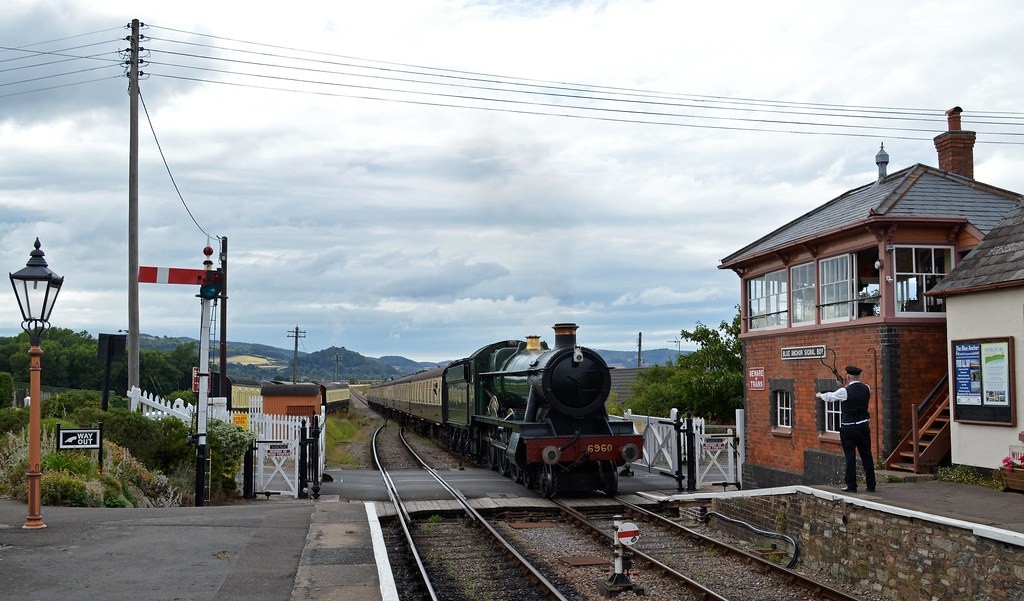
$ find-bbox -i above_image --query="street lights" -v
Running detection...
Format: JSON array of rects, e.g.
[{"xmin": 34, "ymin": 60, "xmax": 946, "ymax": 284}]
[{"xmin": 7, "ymin": 237, "xmax": 65, "ymax": 530}]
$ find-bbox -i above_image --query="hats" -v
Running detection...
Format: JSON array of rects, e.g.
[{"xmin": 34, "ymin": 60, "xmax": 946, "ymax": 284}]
[{"xmin": 845, "ymin": 366, "xmax": 862, "ymax": 376}]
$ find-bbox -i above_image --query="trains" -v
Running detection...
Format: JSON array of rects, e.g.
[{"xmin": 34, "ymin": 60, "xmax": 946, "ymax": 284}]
[{"xmin": 366, "ymin": 323, "xmax": 645, "ymax": 500}]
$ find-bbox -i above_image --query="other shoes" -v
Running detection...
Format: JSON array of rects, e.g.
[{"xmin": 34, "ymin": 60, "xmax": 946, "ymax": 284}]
[
  {"xmin": 841, "ymin": 487, "xmax": 856, "ymax": 493},
  {"xmin": 866, "ymin": 488, "xmax": 875, "ymax": 492}
]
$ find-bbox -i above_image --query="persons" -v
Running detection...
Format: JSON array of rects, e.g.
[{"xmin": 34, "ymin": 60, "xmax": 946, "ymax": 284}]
[{"xmin": 815, "ymin": 366, "xmax": 879, "ymax": 493}]
